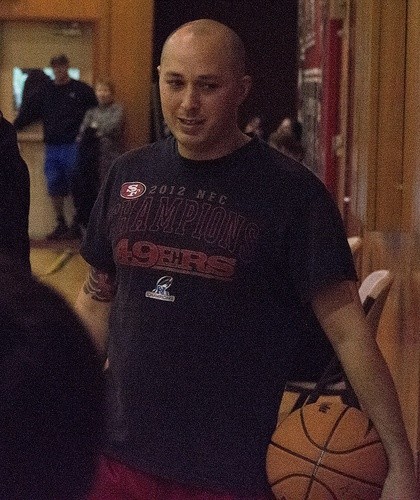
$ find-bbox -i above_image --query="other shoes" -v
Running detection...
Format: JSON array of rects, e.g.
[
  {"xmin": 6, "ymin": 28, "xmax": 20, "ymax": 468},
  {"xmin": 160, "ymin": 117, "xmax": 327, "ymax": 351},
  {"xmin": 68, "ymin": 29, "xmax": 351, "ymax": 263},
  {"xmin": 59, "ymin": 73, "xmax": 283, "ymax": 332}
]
[{"xmin": 45, "ymin": 226, "xmax": 83, "ymax": 239}]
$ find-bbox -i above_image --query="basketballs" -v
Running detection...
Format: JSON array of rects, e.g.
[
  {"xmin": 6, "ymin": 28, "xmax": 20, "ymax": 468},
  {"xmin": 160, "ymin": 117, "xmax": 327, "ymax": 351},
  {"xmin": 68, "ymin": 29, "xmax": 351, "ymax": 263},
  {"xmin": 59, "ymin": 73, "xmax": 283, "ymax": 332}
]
[{"xmin": 266, "ymin": 402, "xmax": 388, "ymax": 500}]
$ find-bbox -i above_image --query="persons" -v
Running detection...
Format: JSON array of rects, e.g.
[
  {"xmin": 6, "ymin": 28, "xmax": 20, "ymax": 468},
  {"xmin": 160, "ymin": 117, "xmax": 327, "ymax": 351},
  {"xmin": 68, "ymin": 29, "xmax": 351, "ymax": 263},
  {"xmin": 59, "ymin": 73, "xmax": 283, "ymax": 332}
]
[
  {"xmin": 0, "ymin": 1, "xmax": 420, "ymax": 500},
  {"xmin": 72, "ymin": 19, "xmax": 420, "ymax": 500}
]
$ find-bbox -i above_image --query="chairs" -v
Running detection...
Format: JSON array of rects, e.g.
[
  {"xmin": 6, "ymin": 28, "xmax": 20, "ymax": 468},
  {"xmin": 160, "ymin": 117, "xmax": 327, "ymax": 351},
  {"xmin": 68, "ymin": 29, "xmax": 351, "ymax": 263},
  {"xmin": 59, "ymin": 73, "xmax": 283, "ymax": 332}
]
[{"xmin": 285, "ymin": 270, "xmax": 394, "ymax": 416}]
